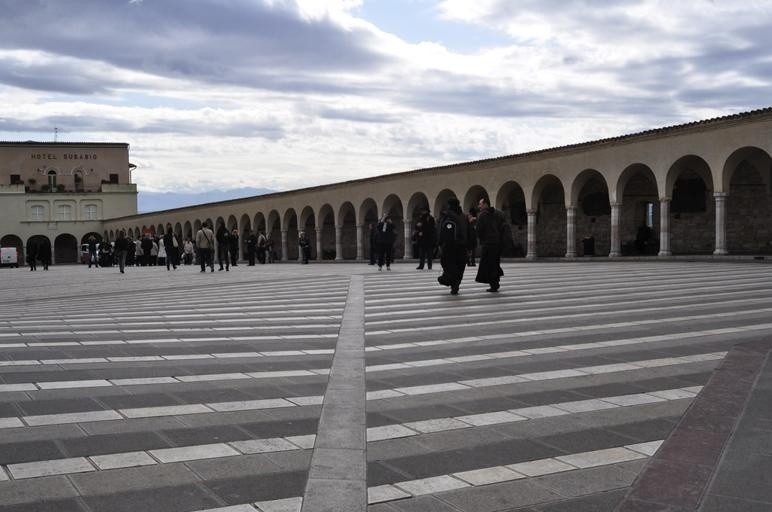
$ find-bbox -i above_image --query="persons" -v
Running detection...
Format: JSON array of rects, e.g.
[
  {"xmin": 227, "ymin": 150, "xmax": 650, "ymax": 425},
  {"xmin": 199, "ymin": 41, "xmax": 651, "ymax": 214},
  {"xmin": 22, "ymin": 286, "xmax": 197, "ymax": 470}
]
[
  {"xmin": 415, "ymin": 197, "xmax": 508, "ymax": 296},
  {"xmin": 297, "ymin": 232, "xmax": 312, "ymax": 264},
  {"xmin": 369, "ymin": 212, "xmax": 396, "ymax": 270},
  {"xmin": 244, "ymin": 229, "xmax": 266, "ymax": 266},
  {"xmin": 88, "ymin": 221, "xmax": 241, "ymax": 274},
  {"xmin": 25, "ymin": 239, "xmax": 51, "ymax": 271}
]
